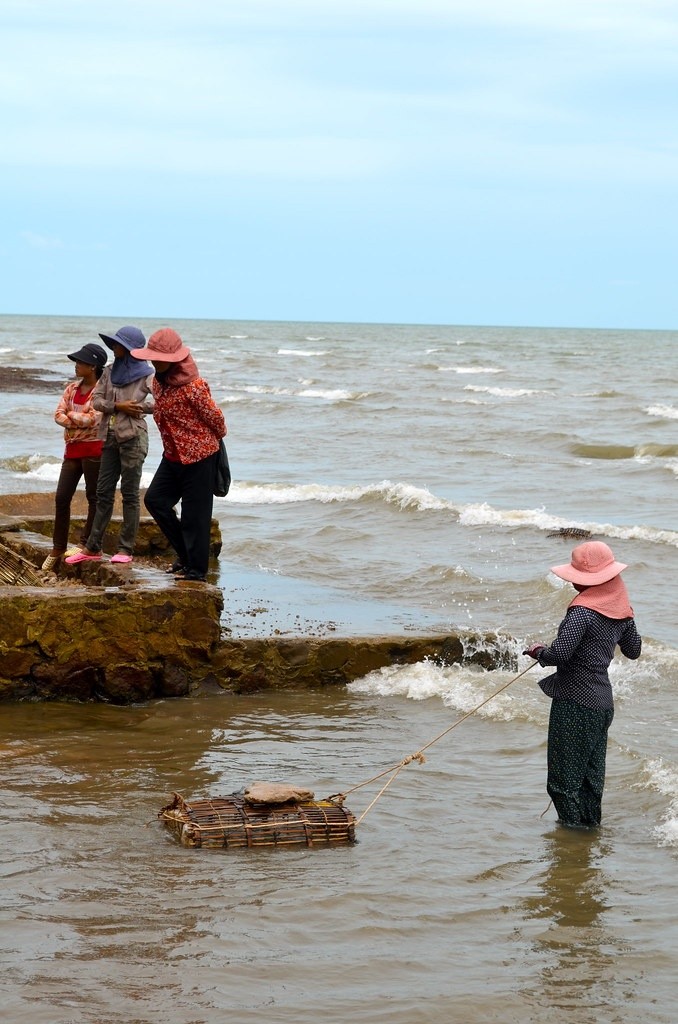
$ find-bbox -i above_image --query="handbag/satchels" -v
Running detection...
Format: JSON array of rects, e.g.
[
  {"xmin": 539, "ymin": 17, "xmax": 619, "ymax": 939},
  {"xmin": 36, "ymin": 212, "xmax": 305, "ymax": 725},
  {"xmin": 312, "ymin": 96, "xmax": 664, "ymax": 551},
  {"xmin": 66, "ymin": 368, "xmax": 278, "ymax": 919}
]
[{"xmin": 214, "ymin": 438, "xmax": 231, "ymax": 497}]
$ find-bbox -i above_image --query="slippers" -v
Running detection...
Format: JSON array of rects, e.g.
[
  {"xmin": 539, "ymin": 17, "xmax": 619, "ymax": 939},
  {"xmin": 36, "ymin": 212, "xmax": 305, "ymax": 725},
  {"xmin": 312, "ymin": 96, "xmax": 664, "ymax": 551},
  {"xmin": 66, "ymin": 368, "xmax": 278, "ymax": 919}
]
[
  {"xmin": 65, "ymin": 545, "xmax": 83, "ymax": 557},
  {"xmin": 111, "ymin": 554, "xmax": 133, "ymax": 562},
  {"xmin": 65, "ymin": 553, "xmax": 102, "ymax": 563},
  {"xmin": 42, "ymin": 551, "xmax": 65, "ymax": 570}
]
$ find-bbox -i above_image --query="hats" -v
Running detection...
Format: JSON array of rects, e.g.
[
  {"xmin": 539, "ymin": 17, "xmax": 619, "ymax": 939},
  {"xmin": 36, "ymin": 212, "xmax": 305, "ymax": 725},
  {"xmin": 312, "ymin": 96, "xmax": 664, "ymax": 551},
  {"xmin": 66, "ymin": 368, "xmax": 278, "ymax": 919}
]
[
  {"xmin": 130, "ymin": 328, "xmax": 190, "ymax": 362},
  {"xmin": 99, "ymin": 326, "xmax": 146, "ymax": 352},
  {"xmin": 550, "ymin": 540, "xmax": 628, "ymax": 586},
  {"xmin": 68, "ymin": 344, "xmax": 107, "ymax": 367}
]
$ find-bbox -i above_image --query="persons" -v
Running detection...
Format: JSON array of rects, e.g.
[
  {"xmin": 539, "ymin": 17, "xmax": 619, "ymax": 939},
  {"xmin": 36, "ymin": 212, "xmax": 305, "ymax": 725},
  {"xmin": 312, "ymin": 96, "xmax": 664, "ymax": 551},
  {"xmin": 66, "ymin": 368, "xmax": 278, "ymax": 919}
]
[
  {"xmin": 130, "ymin": 327, "xmax": 228, "ymax": 580},
  {"xmin": 64, "ymin": 325, "xmax": 156, "ymax": 562},
  {"xmin": 42, "ymin": 343, "xmax": 111, "ymax": 571},
  {"xmin": 522, "ymin": 540, "xmax": 642, "ymax": 828}
]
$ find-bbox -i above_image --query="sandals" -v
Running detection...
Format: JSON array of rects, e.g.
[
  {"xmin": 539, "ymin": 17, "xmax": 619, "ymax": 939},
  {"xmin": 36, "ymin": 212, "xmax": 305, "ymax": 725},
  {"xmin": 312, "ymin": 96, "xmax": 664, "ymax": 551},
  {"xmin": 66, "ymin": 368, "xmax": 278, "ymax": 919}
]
[
  {"xmin": 174, "ymin": 572, "xmax": 206, "ymax": 582},
  {"xmin": 166, "ymin": 557, "xmax": 184, "ymax": 573}
]
